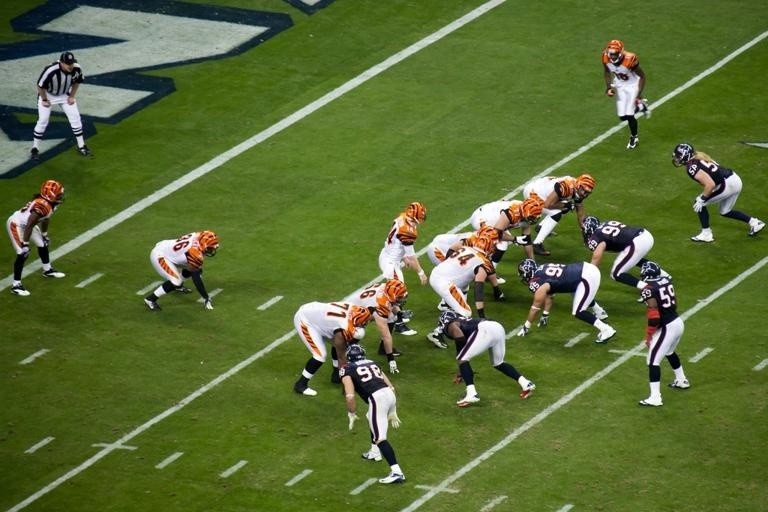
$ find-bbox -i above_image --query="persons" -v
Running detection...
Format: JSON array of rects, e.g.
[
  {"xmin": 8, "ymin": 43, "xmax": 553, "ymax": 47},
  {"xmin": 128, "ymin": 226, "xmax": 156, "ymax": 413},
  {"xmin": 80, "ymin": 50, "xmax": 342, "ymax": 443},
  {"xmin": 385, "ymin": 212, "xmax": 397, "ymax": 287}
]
[
  {"xmin": 144, "ymin": 230, "xmax": 220, "ymax": 311},
  {"xmin": 30, "ymin": 51, "xmax": 94, "ymax": 162},
  {"xmin": 639, "ymin": 262, "xmax": 689, "ymax": 406},
  {"xmin": 427, "ymin": 174, "xmax": 673, "ymax": 407},
  {"xmin": 6, "ymin": 180, "xmax": 66, "ymax": 296},
  {"xmin": 672, "ymin": 143, "xmax": 766, "ymax": 242},
  {"xmin": 293, "ymin": 278, "xmax": 409, "ymax": 484},
  {"xmin": 602, "ymin": 39, "xmax": 652, "ymax": 148},
  {"xmin": 379, "ymin": 202, "xmax": 429, "ymax": 335}
]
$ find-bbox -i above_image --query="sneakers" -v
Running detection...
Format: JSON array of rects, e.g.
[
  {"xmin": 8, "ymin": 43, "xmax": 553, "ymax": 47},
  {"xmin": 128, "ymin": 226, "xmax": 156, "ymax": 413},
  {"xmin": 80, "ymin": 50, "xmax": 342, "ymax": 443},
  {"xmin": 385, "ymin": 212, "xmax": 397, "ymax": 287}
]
[
  {"xmin": 748, "ymin": 219, "xmax": 765, "ymax": 236},
  {"xmin": 389, "ymin": 360, "xmax": 400, "ymax": 375},
  {"xmin": 456, "ymin": 394, "xmax": 480, "ymax": 407},
  {"xmin": 360, "ymin": 447, "xmax": 405, "ymax": 484},
  {"xmin": 78, "ymin": 144, "xmax": 93, "ymax": 157},
  {"xmin": 495, "ymin": 275, "xmax": 506, "ymax": 284},
  {"xmin": 144, "ymin": 299, "xmax": 161, "ymax": 310},
  {"xmin": 177, "ymin": 286, "xmax": 193, "ymax": 292},
  {"xmin": 690, "ymin": 233, "xmax": 714, "ymax": 242},
  {"xmin": 627, "ymin": 134, "xmax": 639, "ymax": 149},
  {"xmin": 669, "ymin": 378, "xmax": 690, "ymax": 389},
  {"xmin": 638, "ymin": 396, "xmax": 663, "ymax": 407},
  {"xmin": 295, "ymin": 382, "xmax": 317, "ymax": 395},
  {"xmin": 31, "ymin": 148, "xmax": 40, "ymax": 161},
  {"xmin": 520, "ymin": 381, "xmax": 536, "ymax": 399},
  {"xmin": 9, "ymin": 268, "xmax": 65, "ymax": 296},
  {"xmin": 427, "ymin": 300, "xmax": 452, "ymax": 350},
  {"xmin": 593, "ymin": 309, "xmax": 616, "ymax": 343},
  {"xmin": 640, "ymin": 98, "xmax": 651, "ymax": 120},
  {"xmin": 378, "ymin": 310, "xmax": 418, "ymax": 357}
]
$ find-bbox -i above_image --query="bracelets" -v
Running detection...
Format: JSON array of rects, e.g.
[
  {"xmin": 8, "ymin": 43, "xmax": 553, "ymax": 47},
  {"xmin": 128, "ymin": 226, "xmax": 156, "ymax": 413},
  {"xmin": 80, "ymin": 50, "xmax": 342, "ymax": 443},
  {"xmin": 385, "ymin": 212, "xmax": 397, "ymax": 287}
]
[{"xmin": 417, "ymin": 269, "xmax": 424, "ymax": 276}]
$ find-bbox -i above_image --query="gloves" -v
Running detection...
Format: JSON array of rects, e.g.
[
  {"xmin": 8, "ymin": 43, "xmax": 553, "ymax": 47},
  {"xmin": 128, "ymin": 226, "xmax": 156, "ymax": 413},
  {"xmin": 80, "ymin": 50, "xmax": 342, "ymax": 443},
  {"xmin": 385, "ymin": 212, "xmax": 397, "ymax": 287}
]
[
  {"xmin": 693, "ymin": 195, "xmax": 707, "ymax": 213},
  {"xmin": 22, "ymin": 241, "xmax": 31, "ymax": 258},
  {"xmin": 41, "ymin": 232, "xmax": 49, "ymax": 246},
  {"xmin": 518, "ymin": 320, "xmax": 533, "ymax": 338},
  {"xmin": 347, "ymin": 412, "xmax": 360, "ymax": 431},
  {"xmin": 204, "ymin": 298, "xmax": 212, "ymax": 310},
  {"xmin": 387, "ymin": 408, "xmax": 402, "ymax": 430},
  {"xmin": 537, "ymin": 314, "xmax": 550, "ymax": 328}
]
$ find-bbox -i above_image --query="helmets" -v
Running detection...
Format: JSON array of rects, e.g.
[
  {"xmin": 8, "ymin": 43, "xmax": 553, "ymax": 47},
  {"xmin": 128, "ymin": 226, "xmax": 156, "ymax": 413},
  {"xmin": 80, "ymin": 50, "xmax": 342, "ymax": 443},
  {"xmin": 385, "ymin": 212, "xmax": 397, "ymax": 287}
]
[
  {"xmin": 40, "ymin": 180, "xmax": 65, "ymax": 204},
  {"xmin": 198, "ymin": 230, "xmax": 221, "ymax": 257},
  {"xmin": 346, "ymin": 279, "xmax": 409, "ymax": 364},
  {"xmin": 438, "ymin": 308, "xmax": 458, "ymax": 337},
  {"xmin": 606, "ymin": 40, "xmax": 624, "ymax": 67},
  {"xmin": 672, "ymin": 143, "xmax": 695, "ymax": 168},
  {"xmin": 640, "ymin": 261, "xmax": 661, "ymax": 281},
  {"xmin": 405, "ymin": 202, "xmax": 426, "ymax": 226},
  {"xmin": 472, "ymin": 173, "xmax": 600, "ymax": 286}
]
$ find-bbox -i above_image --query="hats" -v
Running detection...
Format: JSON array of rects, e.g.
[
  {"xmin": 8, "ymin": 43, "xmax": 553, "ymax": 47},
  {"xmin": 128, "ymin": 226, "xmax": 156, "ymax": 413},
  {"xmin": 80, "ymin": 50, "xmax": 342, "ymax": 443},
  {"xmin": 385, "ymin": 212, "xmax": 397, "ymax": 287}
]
[{"xmin": 60, "ymin": 51, "xmax": 77, "ymax": 64}]
